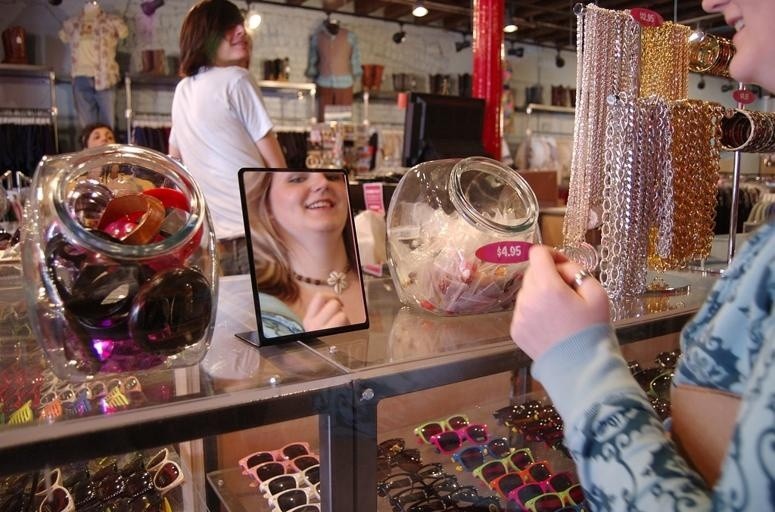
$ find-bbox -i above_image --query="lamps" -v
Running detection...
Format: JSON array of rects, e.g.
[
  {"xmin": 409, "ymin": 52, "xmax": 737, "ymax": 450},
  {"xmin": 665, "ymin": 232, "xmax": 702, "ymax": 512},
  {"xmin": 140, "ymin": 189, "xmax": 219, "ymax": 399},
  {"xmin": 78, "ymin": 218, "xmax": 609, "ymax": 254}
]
[
  {"xmin": 455, "ymin": 32, "xmax": 471, "ymax": 52},
  {"xmin": 556, "ymin": 48, "xmax": 565, "ymax": 68},
  {"xmin": 697, "ymin": 74, "xmax": 707, "ymax": 90},
  {"xmin": 322, "ymin": 10, "xmax": 340, "ymax": 36},
  {"xmin": 411, "ymin": 0, "xmax": 430, "ymax": 18},
  {"xmin": 141, "ymin": 0, "xmax": 166, "ymax": 18},
  {"xmin": 393, "ymin": 23, "xmax": 405, "ymax": 43},
  {"xmin": 508, "ymin": 41, "xmax": 524, "ymax": 58},
  {"xmin": 503, "ymin": 6, "xmax": 518, "ymax": 34},
  {"xmin": 721, "ymin": 78, "xmax": 735, "ymax": 94},
  {"xmin": 242, "ymin": 0, "xmax": 263, "ymax": 31}
]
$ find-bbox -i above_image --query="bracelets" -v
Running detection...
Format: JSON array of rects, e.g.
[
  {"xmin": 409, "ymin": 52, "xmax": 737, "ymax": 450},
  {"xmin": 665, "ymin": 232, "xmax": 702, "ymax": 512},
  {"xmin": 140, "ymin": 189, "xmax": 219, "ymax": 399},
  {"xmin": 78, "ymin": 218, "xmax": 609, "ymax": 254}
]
[
  {"xmin": 44, "ymin": 161, "xmax": 210, "ymax": 371},
  {"xmin": 726, "ymin": 109, "xmax": 774, "ymax": 152},
  {"xmin": 694, "ymin": 33, "xmax": 730, "ymax": 69}
]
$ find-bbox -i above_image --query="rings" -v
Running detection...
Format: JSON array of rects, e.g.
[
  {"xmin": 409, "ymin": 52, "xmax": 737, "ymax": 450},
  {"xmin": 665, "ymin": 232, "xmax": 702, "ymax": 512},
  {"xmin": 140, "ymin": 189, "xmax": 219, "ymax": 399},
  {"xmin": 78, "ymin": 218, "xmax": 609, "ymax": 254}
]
[{"xmin": 574, "ymin": 268, "xmax": 590, "ymax": 291}]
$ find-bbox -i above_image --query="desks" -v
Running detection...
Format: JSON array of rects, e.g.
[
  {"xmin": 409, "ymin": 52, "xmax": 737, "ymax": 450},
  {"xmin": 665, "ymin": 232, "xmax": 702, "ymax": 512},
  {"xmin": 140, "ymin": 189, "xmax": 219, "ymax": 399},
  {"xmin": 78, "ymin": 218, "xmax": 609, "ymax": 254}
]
[{"xmin": 0, "ymin": 228, "xmax": 353, "ymax": 510}]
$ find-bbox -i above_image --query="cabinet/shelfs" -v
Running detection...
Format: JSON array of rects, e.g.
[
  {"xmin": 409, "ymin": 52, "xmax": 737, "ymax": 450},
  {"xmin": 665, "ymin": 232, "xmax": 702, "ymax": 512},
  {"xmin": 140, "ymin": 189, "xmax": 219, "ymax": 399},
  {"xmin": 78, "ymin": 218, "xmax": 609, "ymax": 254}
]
[
  {"xmin": 515, "ymin": 97, "xmax": 580, "ymax": 175},
  {"xmin": 225, "ymin": 229, "xmax": 775, "ymax": 512},
  {"xmin": 0, "ymin": 64, "xmax": 61, "ymax": 193},
  {"xmin": 125, "ymin": 70, "xmax": 318, "ymax": 183}
]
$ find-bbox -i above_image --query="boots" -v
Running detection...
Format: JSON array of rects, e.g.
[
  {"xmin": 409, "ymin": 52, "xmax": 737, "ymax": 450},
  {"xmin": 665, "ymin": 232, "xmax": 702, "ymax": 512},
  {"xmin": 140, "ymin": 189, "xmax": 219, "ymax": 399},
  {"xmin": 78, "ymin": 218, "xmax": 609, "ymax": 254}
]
[
  {"xmin": 276, "ymin": 57, "xmax": 291, "ymax": 82},
  {"xmin": 359, "ymin": 64, "xmax": 371, "ymax": 90},
  {"xmin": 373, "ymin": 65, "xmax": 384, "ymax": 92},
  {"xmin": 262, "ymin": 60, "xmax": 275, "ymax": 80},
  {"xmin": 146, "ymin": 50, "xmax": 166, "ymax": 76},
  {"xmin": 559, "ymin": 84, "xmax": 569, "ymax": 107},
  {"xmin": 432, "ymin": 74, "xmax": 440, "ymax": 93},
  {"xmin": 394, "ymin": 73, "xmax": 404, "ymax": 90},
  {"xmin": 532, "ymin": 86, "xmax": 543, "ymax": 104},
  {"xmin": 406, "ymin": 73, "xmax": 416, "ymax": 93},
  {"xmin": 8, "ymin": 25, "xmax": 28, "ymax": 64},
  {"xmin": 1, "ymin": 32, "xmax": 11, "ymax": 63},
  {"xmin": 438, "ymin": 76, "xmax": 449, "ymax": 95},
  {"xmin": 551, "ymin": 85, "xmax": 561, "ymax": 106},
  {"xmin": 137, "ymin": 50, "xmax": 153, "ymax": 75},
  {"xmin": 166, "ymin": 53, "xmax": 178, "ymax": 77},
  {"xmin": 524, "ymin": 88, "xmax": 533, "ymax": 104}
]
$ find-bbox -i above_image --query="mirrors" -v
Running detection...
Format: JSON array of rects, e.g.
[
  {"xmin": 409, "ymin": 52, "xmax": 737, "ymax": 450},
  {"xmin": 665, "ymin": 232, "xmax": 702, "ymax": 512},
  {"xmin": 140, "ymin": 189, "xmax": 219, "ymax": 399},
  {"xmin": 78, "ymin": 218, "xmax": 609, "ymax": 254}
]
[{"xmin": 234, "ymin": 168, "xmax": 371, "ymax": 349}]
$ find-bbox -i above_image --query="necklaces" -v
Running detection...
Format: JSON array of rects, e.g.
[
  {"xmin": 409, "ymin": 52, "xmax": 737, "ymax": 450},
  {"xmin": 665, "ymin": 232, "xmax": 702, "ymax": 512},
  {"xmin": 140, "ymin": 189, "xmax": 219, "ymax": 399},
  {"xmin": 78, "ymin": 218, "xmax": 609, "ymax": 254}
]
[
  {"xmin": 565, "ymin": 2, "xmax": 720, "ymax": 309},
  {"xmin": 289, "ymin": 267, "xmax": 350, "ymax": 289},
  {"xmin": 608, "ymin": 297, "xmax": 672, "ymax": 322}
]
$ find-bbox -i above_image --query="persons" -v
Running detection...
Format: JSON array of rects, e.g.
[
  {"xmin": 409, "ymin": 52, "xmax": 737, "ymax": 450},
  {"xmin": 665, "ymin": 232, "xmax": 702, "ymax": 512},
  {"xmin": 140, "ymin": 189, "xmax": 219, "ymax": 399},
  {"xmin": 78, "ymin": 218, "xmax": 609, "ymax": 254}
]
[
  {"xmin": 305, "ymin": 20, "xmax": 363, "ymax": 121},
  {"xmin": 511, "ymin": 0, "xmax": 775, "ymax": 512},
  {"xmin": 59, "ymin": 2, "xmax": 130, "ymax": 144},
  {"xmin": 81, "ymin": 125, "xmax": 116, "ymax": 146},
  {"xmin": 241, "ymin": 170, "xmax": 366, "ymax": 336},
  {"xmin": 168, "ymin": 3, "xmax": 287, "ymax": 276}
]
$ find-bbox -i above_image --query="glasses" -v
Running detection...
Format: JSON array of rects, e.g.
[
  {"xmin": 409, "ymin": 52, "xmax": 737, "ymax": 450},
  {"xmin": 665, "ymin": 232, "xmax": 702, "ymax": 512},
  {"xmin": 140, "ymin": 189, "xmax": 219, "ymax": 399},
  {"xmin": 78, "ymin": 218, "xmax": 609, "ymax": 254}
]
[
  {"xmin": 1, "ymin": 387, "xmax": 22, "ymax": 410},
  {"xmin": 270, "ymin": 481, "xmax": 321, "ymax": 512},
  {"xmin": 446, "ymin": 496, "xmax": 500, "ymax": 512},
  {"xmin": 554, "ymin": 439, "xmax": 570, "ymax": 458},
  {"xmin": 508, "ymin": 470, "xmax": 576, "ymax": 510},
  {"xmin": 556, "ymin": 500, "xmax": 592, "ymax": 512},
  {"xmin": 376, "ymin": 438, "xmax": 404, "ymax": 462},
  {"xmin": 526, "ymin": 414, "xmax": 561, "ymax": 437},
  {"xmin": 629, "ymin": 360, "xmax": 640, "ymax": 375},
  {"xmin": 511, "ymin": 405, "xmax": 557, "ymax": 429},
  {"xmin": 146, "ymin": 448, "xmax": 184, "ymax": 494},
  {"xmin": 42, "ymin": 367, "xmax": 57, "ymax": 385},
  {"xmin": 472, "ymin": 448, "xmax": 534, "ymax": 487},
  {"xmin": 654, "ymin": 348, "xmax": 680, "ymax": 369},
  {"xmin": 91, "ymin": 381, "xmax": 108, "ymax": 398},
  {"xmin": 0, "ymin": 473, "xmax": 27, "ymax": 512},
  {"xmin": 121, "ymin": 458, "xmax": 152, "ymax": 497},
  {"xmin": 40, "ymin": 399, "xmax": 63, "ymax": 418},
  {"xmin": 104, "ymin": 497, "xmax": 127, "ymax": 512},
  {"xmin": 91, "ymin": 463, "xmax": 125, "ymax": 501},
  {"xmin": 540, "ymin": 422, "xmax": 563, "ymax": 446},
  {"xmin": 23, "ymin": 468, "xmax": 40, "ymax": 512},
  {"xmin": 376, "ymin": 463, "xmax": 444, "ymax": 501},
  {"xmin": 131, "ymin": 494, "xmax": 157, "ymax": 512},
  {"xmin": 97, "ymin": 385, "xmax": 128, "ymax": 413},
  {"xmin": 408, "ymin": 487, "xmax": 478, "ymax": 511},
  {"xmin": 648, "ymin": 372, "xmax": 673, "ymax": 401},
  {"xmin": 71, "ymin": 381, "xmax": 93, "ymax": 400},
  {"xmin": 413, "ymin": 415, "xmax": 470, "ymax": 444},
  {"xmin": 494, "ymin": 400, "xmax": 543, "ymax": 426},
  {"xmin": 69, "ymin": 388, "xmax": 91, "ymax": 417},
  {"xmin": 7, "ymin": 400, "xmax": 32, "ymax": 424},
  {"xmin": 376, "ymin": 448, "xmax": 423, "ymax": 482},
  {"xmin": 57, "ymin": 380, "xmax": 75, "ymax": 403},
  {"xmin": 259, "ymin": 465, "xmax": 320, "ymax": 496},
  {"xmin": 248, "ymin": 455, "xmax": 320, "ymax": 484},
  {"xmin": 14, "ymin": 311, "xmax": 31, "ymax": 337},
  {"xmin": 239, "ymin": 442, "xmax": 309, "ymax": 470},
  {"xmin": 35, "ymin": 468, "xmax": 75, "ymax": 511},
  {"xmin": 286, "ymin": 503, "xmax": 320, "ymax": 511},
  {"xmin": 450, "ymin": 437, "xmax": 511, "ymax": 471},
  {"xmin": 24, "ymin": 384, "xmax": 39, "ymax": 410},
  {"xmin": 525, "ymin": 483, "xmax": 587, "ymax": 512},
  {"xmin": 63, "ymin": 465, "xmax": 95, "ymax": 505},
  {"xmin": 390, "ymin": 476, "xmax": 459, "ymax": 512},
  {"xmin": 124, "ymin": 376, "xmax": 140, "ymax": 392},
  {"xmin": 489, "ymin": 461, "xmax": 553, "ymax": 498},
  {"xmin": 108, "ymin": 379, "xmax": 124, "ymax": 394},
  {"xmin": 430, "ymin": 424, "xmax": 490, "ymax": 454},
  {"xmin": 40, "ymin": 383, "xmax": 57, "ymax": 407}
]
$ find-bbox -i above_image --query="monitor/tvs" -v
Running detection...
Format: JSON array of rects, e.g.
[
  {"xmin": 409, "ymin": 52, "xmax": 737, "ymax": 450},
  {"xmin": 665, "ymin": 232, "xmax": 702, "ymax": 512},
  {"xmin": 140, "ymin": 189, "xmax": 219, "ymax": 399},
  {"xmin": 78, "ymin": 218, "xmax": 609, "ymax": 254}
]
[{"xmin": 402, "ymin": 90, "xmax": 486, "ymax": 169}]
